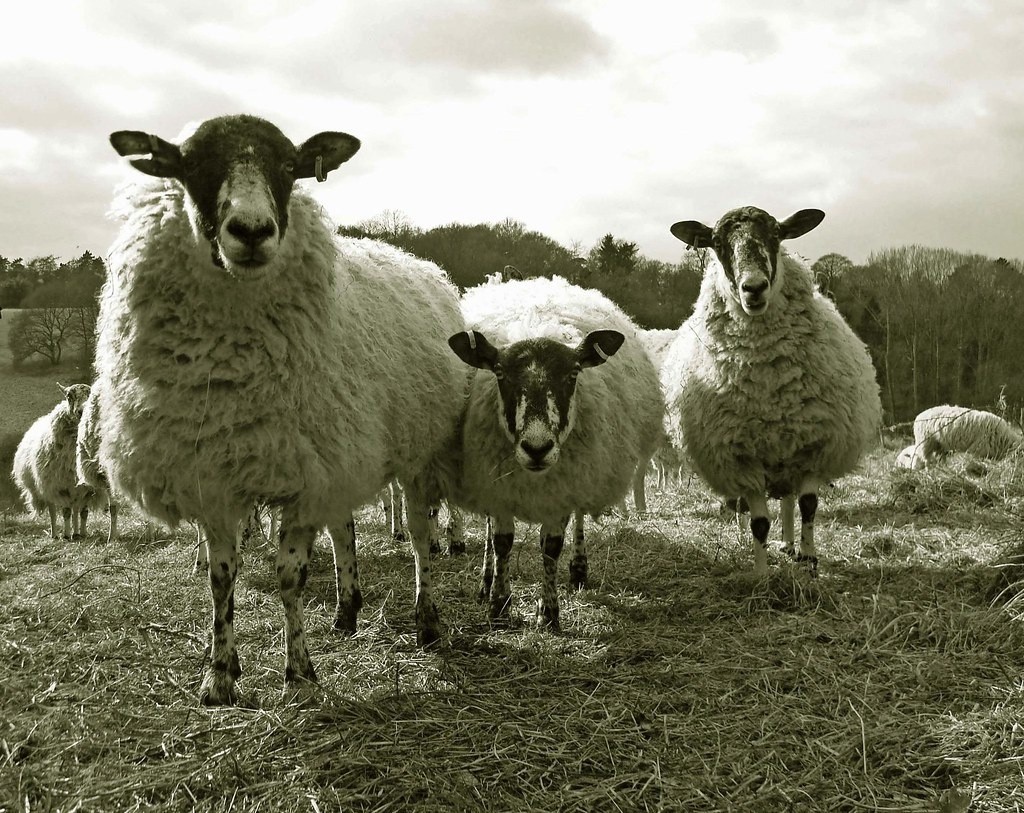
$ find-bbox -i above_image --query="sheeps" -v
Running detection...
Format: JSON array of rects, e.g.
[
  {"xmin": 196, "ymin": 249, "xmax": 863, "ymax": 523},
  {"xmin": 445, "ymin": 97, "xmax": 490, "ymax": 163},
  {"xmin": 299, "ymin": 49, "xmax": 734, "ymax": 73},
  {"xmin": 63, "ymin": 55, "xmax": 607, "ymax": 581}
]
[
  {"xmin": 12, "ymin": 379, "xmax": 120, "ymax": 543},
  {"xmin": 449, "ymin": 277, "xmax": 661, "ymax": 631},
  {"xmin": 913, "ymin": 405, "xmax": 1020, "ymax": 465},
  {"xmin": 659, "ymin": 206, "xmax": 880, "ymax": 576},
  {"xmin": 87, "ymin": 115, "xmax": 462, "ymax": 702}
]
